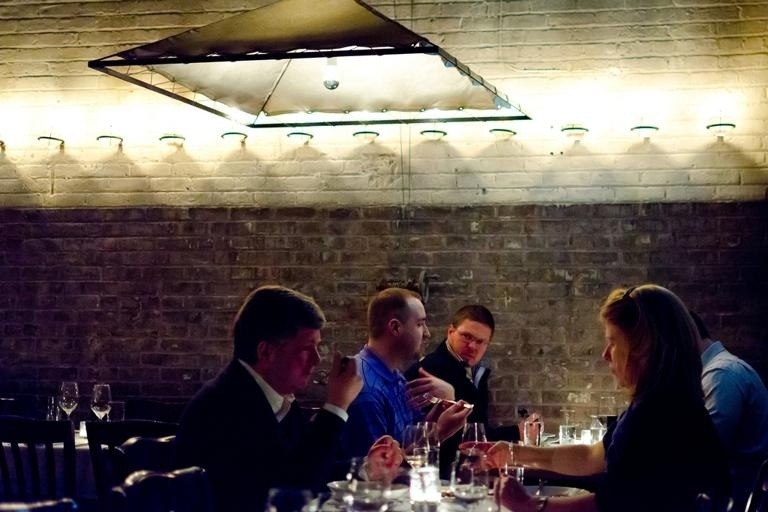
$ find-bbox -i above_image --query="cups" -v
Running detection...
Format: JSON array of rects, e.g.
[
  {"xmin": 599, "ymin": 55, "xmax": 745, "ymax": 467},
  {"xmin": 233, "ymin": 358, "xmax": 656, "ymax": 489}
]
[
  {"xmin": 266, "ymin": 422, "xmax": 532, "ymax": 512},
  {"xmin": 79, "ymin": 421, "xmax": 87, "ymax": 437},
  {"xmin": 107, "ymin": 402, "xmax": 125, "ymax": 422},
  {"xmin": 559, "ymin": 409, "xmax": 607, "ymax": 446}
]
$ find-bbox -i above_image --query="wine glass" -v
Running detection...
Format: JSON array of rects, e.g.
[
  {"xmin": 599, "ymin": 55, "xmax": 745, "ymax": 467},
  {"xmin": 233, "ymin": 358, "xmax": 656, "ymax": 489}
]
[
  {"xmin": 91, "ymin": 383, "xmax": 111, "ymax": 420},
  {"xmin": 56, "ymin": 381, "xmax": 79, "ymax": 420}
]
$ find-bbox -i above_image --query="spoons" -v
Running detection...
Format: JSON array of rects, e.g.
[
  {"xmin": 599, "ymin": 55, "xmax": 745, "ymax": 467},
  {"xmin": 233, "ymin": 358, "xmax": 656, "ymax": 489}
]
[{"xmin": 313, "ymin": 357, "xmax": 347, "ymax": 387}]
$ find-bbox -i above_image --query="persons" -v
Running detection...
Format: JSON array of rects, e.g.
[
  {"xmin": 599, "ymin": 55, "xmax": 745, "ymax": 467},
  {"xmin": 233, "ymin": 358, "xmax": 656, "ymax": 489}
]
[
  {"xmin": 168, "ymin": 282, "xmax": 406, "ymax": 511},
  {"xmin": 454, "ymin": 266, "xmax": 739, "ymax": 510},
  {"xmin": 403, "ymin": 302, "xmax": 545, "ymax": 478},
  {"xmin": 330, "ymin": 285, "xmax": 477, "ymax": 485},
  {"xmin": 678, "ymin": 303, "xmax": 768, "ymax": 512}
]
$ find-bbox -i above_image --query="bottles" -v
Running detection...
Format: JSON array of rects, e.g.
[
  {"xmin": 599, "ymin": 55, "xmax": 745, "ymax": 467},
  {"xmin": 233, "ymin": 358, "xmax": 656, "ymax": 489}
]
[{"xmin": 45, "ymin": 395, "xmax": 57, "ymax": 421}]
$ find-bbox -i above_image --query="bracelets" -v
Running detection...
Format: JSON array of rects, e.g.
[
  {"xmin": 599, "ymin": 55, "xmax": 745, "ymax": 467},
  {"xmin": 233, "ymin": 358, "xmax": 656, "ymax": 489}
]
[{"xmin": 508, "ymin": 440, "xmax": 516, "ymax": 467}]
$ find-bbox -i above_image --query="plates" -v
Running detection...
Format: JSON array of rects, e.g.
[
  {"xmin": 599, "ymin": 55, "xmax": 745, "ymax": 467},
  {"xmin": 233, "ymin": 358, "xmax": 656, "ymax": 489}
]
[
  {"xmin": 524, "ymin": 484, "xmax": 590, "ymax": 498},
  {"xmin": 540, "ymin": 432, "xmax": 556, "ymax": 442}
]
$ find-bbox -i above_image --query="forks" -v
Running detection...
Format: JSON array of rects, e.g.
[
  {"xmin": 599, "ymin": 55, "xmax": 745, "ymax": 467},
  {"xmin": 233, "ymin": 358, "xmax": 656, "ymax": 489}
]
[{"xmin": 423, "ymin": 390, "xmax": 476, "ymax": 410}]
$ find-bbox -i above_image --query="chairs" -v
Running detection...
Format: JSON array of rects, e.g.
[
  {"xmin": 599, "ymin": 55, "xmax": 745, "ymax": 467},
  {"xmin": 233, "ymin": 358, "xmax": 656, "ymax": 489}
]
[
  {"xmin": 86, "ymin": 420, "xmax": 176, "ymax": 512},
  {"xmin": 0, "ymin": 414, "xmax": 81, "ymax": 512},
  {"xmin": 124, "ymin": 397, "xmax": 185, "ymax": 423},
  {"xmin": 695, "ymin": 449, "xmax": 768, "ymax": 512}
]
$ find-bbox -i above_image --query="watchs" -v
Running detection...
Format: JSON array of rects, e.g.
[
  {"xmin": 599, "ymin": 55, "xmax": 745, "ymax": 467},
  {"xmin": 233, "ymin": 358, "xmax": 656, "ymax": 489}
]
[{"xmin": 533, "ymin": 494, "xmax": 551, "ymax": 511}]
